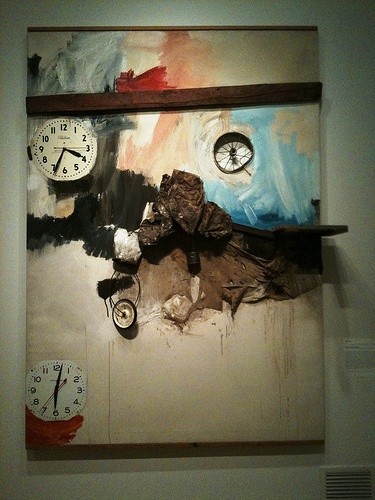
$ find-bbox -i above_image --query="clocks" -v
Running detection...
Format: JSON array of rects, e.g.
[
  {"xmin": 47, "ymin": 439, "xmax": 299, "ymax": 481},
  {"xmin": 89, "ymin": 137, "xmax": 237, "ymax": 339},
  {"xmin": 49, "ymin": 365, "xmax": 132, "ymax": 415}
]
[
  {"xmin": 30, "ymin": 115, "xmax": 101, "ymax": 182},
  {"xmin": 24, "ymin": 358, "xmax": 88, "ymax": 422}
]
